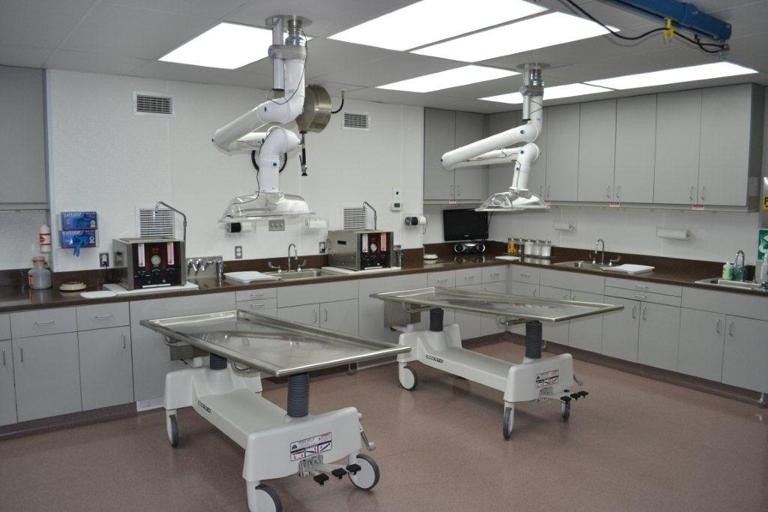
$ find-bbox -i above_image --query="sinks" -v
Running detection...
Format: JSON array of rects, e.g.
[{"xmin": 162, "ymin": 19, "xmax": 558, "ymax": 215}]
[{"xmin": 267, "ymin": 270, "xmax": 349, "ymax": 280}]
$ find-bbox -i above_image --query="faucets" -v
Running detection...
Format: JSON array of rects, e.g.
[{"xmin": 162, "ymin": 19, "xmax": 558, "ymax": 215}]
[
  {"xmin": 288, "ymin": 244, "xmax": 297, "ymax": 272},
  {"xmin": 362, "ymin": 201, "xmax": 377, "ymax": 230}
]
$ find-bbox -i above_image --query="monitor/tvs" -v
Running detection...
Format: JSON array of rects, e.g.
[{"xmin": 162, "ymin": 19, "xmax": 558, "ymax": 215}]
[{"xmin": 443, "ymin": 208, "xmax": 490, "ymax": 241}]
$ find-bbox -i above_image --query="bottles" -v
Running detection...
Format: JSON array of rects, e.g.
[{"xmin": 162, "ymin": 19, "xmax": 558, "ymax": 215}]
[
  {"xmin": 720, "ymin": 257, "xmax": 743, "ymax": 281},
  {"xmin": 39, "ymin": 222, "xmax": 52, "ymax": 253},
  {"xmin": 505, "ymin": 237, "xmax": 551, "ymax": 257},
  {"xmin": 26, "ymin": 256, "xmax": 51, "ymax": 290}
]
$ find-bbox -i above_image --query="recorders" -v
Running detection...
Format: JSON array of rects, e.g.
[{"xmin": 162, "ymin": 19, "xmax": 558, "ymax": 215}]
[{"xmin": 454, "ymin": 242, "xmax": 487, "ymax": 254}]
